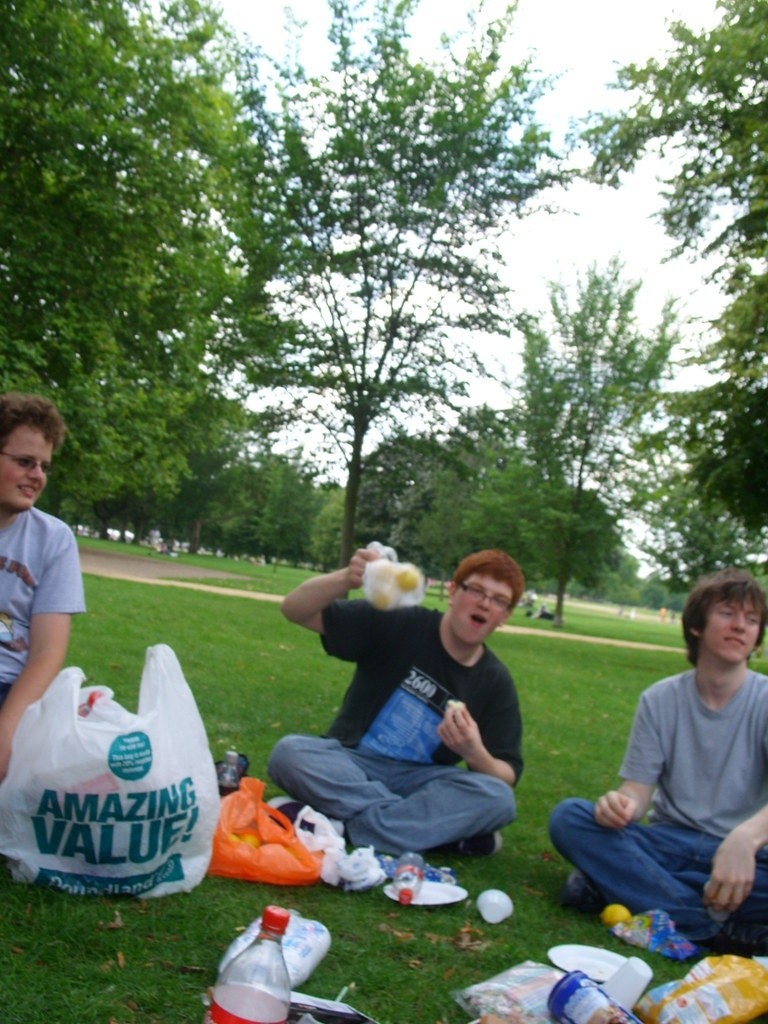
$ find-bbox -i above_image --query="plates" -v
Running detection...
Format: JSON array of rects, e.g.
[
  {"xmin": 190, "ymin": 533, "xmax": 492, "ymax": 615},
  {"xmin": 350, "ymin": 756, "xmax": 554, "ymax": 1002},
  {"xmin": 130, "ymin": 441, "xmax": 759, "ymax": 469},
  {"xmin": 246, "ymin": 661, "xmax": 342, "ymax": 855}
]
[
  {"xmin": 383, "ymin": 880, "xmax": 468, "ymax": 906},
  {"xmin": 547, "ymin": 944, "xmax": 627, "ymax": 983}
]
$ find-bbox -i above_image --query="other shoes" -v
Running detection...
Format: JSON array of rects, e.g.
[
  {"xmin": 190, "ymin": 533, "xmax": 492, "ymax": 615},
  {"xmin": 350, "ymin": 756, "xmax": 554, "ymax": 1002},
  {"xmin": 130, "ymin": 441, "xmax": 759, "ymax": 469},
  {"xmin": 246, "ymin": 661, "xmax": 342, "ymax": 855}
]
[
  {"xmin": 425, "ymin": 829, "xmax": 504, "ymax": 857},
  {"xmin": 558, "ymin": 868, "xmax": 608, "ymax": 915}
]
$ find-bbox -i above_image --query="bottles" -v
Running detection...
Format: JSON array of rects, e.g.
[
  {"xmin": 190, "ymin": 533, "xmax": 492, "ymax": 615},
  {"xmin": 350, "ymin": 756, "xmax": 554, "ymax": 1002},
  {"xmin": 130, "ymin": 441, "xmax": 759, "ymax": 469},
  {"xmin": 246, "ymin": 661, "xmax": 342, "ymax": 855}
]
[
  {"xmin": 394, "ymin": 852, "xmax": 424, "ymax": 905},
  {"xmin": 217, "ymin": 745, "xmax": 239, "ymax": 799},
  {"xmin": 204, "ymin": 905, "xmax": 291, "ymax": 1024}
]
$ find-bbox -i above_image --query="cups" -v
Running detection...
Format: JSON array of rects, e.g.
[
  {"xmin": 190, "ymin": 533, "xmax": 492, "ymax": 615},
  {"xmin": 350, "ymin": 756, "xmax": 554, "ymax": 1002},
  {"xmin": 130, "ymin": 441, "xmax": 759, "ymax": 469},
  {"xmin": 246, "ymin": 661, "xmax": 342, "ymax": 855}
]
[
  {"xmin": 477, "ymin": 889, "xmax": 514, "ymax": 924},
  {"xmin": 602, "ymin": 955, "xmax": 654, "ymax": 1010}
]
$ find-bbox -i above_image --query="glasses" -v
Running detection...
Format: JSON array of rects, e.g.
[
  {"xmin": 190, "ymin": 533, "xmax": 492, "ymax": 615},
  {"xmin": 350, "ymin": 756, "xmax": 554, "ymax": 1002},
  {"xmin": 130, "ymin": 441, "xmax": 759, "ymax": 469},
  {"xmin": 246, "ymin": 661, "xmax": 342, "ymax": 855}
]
[
  {"xmin": 458, "ymin": 581, "xmax": 513, "ymax": 612},
  {"xmin": 2, "ymin": 451, "xmax": 52, "ymax": 476}
]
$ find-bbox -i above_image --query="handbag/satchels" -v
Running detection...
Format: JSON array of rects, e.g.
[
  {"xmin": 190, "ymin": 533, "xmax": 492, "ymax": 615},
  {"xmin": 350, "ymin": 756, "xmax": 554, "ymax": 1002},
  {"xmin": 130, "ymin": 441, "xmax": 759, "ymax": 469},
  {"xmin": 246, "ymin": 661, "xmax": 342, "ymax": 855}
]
[
  {"xmin": 206, "ymin": 776, "xmax": 325, "ymax": 886},
  {"xmin": 0, "ymin": 644, "xmax": 220, "ymax": 900}
]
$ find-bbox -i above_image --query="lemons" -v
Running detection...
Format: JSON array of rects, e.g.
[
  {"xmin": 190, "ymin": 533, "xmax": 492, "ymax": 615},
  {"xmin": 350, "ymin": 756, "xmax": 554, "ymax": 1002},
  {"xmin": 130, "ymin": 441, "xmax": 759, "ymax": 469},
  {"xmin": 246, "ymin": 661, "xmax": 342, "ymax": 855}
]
[
  {"xmin": 221, "ymin": 819, "xmax": 305, "ymax": 874},
  {"xmin": 602, "ymin": 902, "xmax": 631, "ymax": 930},
  {"xmin": 372, "ymin": 564, "xmax": 419, "ymax": 610}
]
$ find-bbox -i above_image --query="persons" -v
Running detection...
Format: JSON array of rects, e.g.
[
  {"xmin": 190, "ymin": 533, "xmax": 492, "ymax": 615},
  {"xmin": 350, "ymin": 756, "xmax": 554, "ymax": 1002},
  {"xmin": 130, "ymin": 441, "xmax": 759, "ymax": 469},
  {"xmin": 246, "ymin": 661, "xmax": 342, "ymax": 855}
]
[
  {"xmin": 524, "ymin": 591, "xmax": 565, "ymax": 626},
  {"xmin": 549, "ymin": 568, "xmax": 768, "ymax": 957},
  {"xmin": 618, "ymin": 604, "xmax": 675, "ymax": 624},
  {"xmin": 148, "ymin": 525, "xmax": 179, "ymax": 558},
  {"xmin": 0, "ymin": 393, "xmax": 87, "ymax": 785},
  {"xmin": 265, "ymin": 548, "xmax": 524, "ymax": 858}
]
